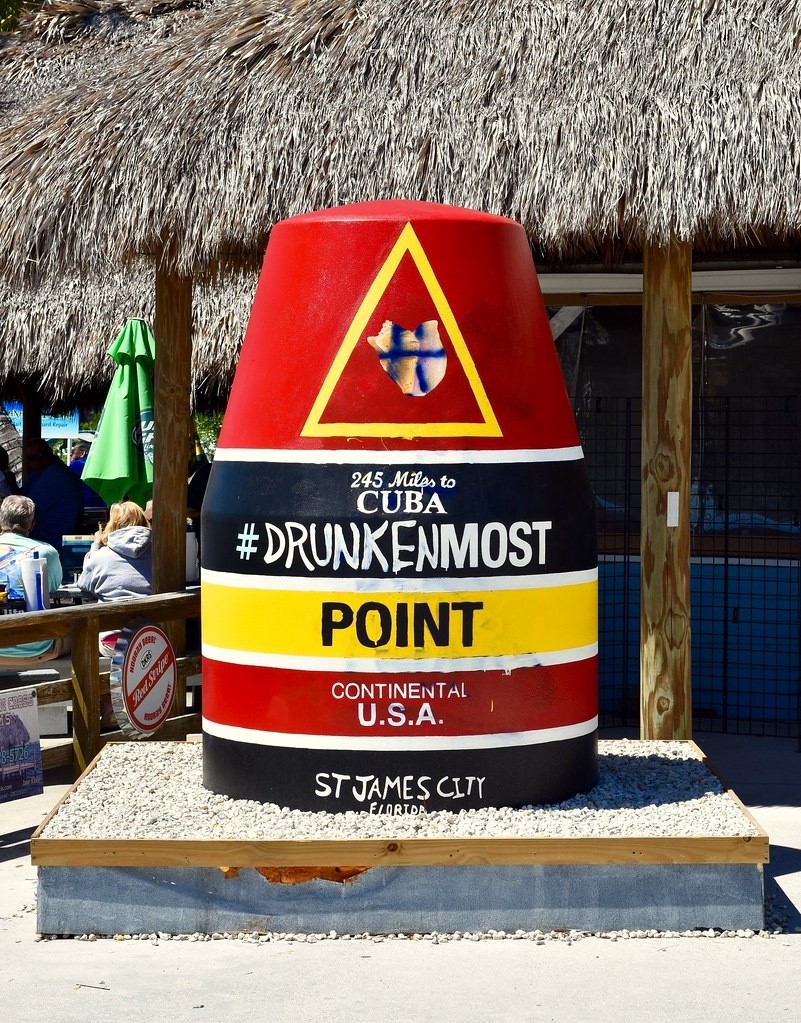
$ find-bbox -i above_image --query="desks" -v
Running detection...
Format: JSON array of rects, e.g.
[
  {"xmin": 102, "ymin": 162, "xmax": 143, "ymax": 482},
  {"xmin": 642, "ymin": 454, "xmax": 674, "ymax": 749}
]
[{"xmin": 48, "ymin": 586, "xmax": 100, "ymax": 603}]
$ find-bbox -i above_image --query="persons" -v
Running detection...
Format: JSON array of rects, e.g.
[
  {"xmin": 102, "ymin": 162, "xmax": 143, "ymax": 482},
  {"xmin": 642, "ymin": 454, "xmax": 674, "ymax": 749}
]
[
  {"xmin": 0, "ymin": 495, "xmax": 64, "ymax": 667},
  {"xmin": 77, "ymin": 499, "xmax": 199, "ymax": 728},
  {"xmin": 67, "ymin": 443, "xmax": 106, "ymax": 507},
  {"xmin": 19, "ymin": 438, "xmax": 82, "ymax": 556},
  {"xmin": 0, "ymin": 445, "xmax": 22, "ymax": 498}
]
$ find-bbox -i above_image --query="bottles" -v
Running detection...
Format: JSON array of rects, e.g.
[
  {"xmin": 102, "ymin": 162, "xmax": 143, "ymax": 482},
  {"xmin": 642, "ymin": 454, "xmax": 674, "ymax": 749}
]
[
  {"xmin": 21, "ymin": 552, "xmax": 51, "ymax": 612},
  {"xmin": 186, "ymin": 525, "xmax": 200, "ymax": 582}
]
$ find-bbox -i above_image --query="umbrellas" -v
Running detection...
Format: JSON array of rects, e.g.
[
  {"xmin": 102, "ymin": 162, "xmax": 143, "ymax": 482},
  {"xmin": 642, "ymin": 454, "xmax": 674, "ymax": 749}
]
[{"xmin": 80, "ymin": 315, "xmax": 206, "ymax": 511}]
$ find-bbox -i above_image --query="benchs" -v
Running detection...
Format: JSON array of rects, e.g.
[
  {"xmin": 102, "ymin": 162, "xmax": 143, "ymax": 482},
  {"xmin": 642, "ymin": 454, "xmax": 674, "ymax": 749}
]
[{"xmin": 0, "ymin": 647, "xmax": 111, "ymax": 676}]
